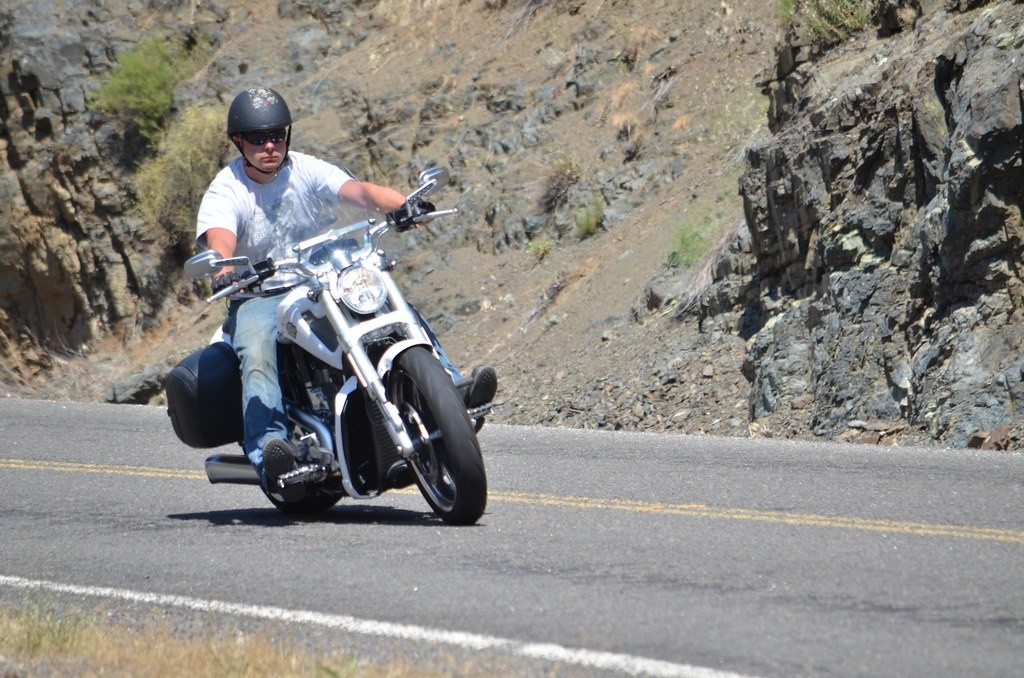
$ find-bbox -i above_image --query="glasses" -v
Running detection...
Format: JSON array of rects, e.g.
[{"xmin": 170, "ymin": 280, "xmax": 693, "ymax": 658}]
[{"xmin": 242, "ymin": 129, "xmax": 285, "ymax": 144}]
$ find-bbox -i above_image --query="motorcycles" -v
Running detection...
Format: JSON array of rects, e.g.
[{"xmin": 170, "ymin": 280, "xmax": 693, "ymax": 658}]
[{"xmin": 182, "ymin": 166, "xmax": 512, "ymax": 528}]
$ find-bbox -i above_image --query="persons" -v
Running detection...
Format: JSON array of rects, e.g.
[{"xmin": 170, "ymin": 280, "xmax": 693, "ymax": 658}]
[{"xmin": 196, "ymin": 90, "xmax": 498, "ymax": 497}]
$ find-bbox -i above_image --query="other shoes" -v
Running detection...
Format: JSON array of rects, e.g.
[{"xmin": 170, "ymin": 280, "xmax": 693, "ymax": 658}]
[
  {"xmin": 263, "ymin": 438, "xmax": 307, "ymax": 503},
  {"xmin": 458, "ymin": 365, "xmax": 498, "ymax": 434}
]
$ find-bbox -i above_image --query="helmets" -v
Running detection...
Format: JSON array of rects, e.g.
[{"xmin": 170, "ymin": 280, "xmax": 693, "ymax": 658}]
[{"xmin": 227, "ymin": 88, "xmax": 292, "ymax": 133}]
